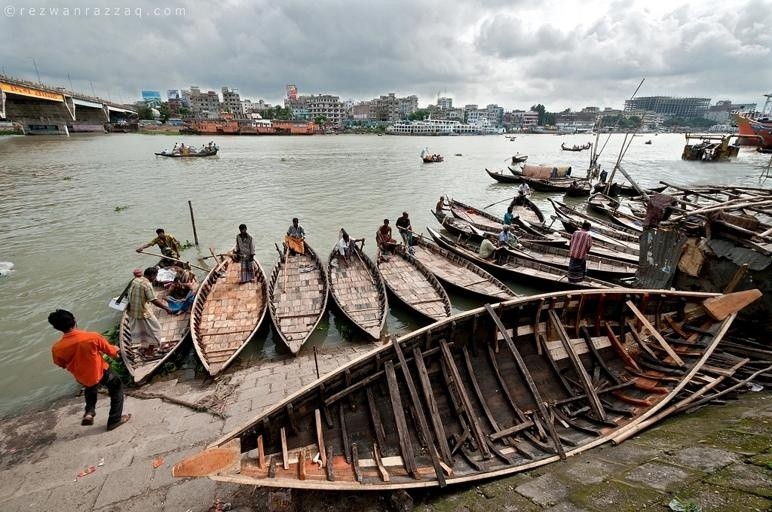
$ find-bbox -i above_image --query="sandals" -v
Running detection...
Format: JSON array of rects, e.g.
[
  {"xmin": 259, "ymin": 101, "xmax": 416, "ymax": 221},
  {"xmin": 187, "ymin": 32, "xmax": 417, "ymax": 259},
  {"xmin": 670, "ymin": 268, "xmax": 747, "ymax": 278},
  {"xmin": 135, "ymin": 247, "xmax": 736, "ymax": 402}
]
[
  {"xmin": 80, "ymin": 413, "xmax": 94, "ymax": 426},
  {"xmin": 106, "ymin": 414, "xmax": 131, "ymax": 431}
]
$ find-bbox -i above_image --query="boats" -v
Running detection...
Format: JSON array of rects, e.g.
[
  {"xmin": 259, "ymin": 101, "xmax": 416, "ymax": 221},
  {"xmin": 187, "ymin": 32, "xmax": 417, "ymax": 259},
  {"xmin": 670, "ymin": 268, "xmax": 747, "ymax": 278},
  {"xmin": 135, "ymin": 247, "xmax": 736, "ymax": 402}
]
[
  {"xmin": 507, "ymin": 164, "xmax": 591, "ymax": 182},
  {"xmin": 507, "ymin": 195, "xmax": 546, "ymax": 237},
  {"xmin": 169, "ymin": 288, "xmax": 763, "ymax": 492},
  {"xmin": 550, "ymin": 199, "xmax": 642, "ymax": 241},
  {"xmin": 512, "ymin": 155, "xmax": 527, "ymax": 163},
  {"xmin": 481, "ymin": 166, "xmax": 582, "ymax": 185},
  {"xmin": 587, "ymin": 180, "xmax": 668, "ymax": 196},
  {"xmin": 599, "ymin": 201, "xmax": 649, "ymax": 234},
  {"xmin": 420, "ymin": 154, "xmax": 445, "ymax": 163},
  {"xmin": 155, "ymin": 145, "xmax": 219, "ymax": 157},
  {"xmin": 425, "ymin": 224, "xmax": 625, "ymax": 292},
  {"xmin": 447, "ymin": 199, "xmax": 567, "ymax": 248},
  {"xmin": 627, "ymin": 203, "xmax": 646, "ymax": 218},
  {"xmin": 377, "ymin": 239, "xmax": 452, "ymax": 323},
  {"xmin": 120, "ymin": 260, "xmax": 198, "ymax": 387},
  {"xmin": 555, "ymin": 210, "xmax": 644, "ymax": 253},
  {"xmin": 525, "ymin": 180, "xmax": 594, "ymax": 192},
  {"xmin": 401, "ymin": 236, "xmax": 521, "ymax": 302},
  {"xmin": 729, "ymin": 93, "xmax": 772, "ymax": 149},
  {"xmin": 190, "ymin": 245, "xmax": 268, "ymax": 376},
  {"xmin": 587, "ymin": 191, "xmax": 620, "ymax": 215},
  {"xmin": 269, "ymin": 239, "xmax": 329, "ymax": 355},
  {"xmin": 563, "ymin": 142, "xmax": 591, "ymax": 151},
  {"xmin": 431, "ymin": 210, "xmax": 639, "ymax": 278},
  {"xmin": 525, "ymin": 219, "xmax": 644, "ymax": 263},
  {"xmin": 325, "ymin": 228, "xmax": 386, "ymax": 341}
]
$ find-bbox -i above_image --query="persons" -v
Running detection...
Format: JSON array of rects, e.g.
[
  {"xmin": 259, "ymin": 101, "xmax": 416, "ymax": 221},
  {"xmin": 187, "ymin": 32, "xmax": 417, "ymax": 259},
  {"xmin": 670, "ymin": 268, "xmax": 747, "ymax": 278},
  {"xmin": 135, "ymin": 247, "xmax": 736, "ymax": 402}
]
[
  {"xmin": 497, "ymin": 223, "xmax": 526, "ymax": 251},
  {"xmin": 135, "ymin": 227, "xmax": 182, "ymax": 262},
  {"xmin": 566, "ymin": 220, "xmax": 594, "ymax": 283},
  {"xmin": 517, "ymin": 181, "xmax": 532, "ymax": 197},
  {"xmin": 114, "ymin": 268, "xmax": 143, "ymax": 317},
  {"xmin": 126, "ymin": 266, "xmax": 175, "ymax": 364},
  {"xmin": 165, "ymin": 278, "xmax": 196, "ymax": 316},
  {"xmin": 284, "ymin": 217, "xmax": 307, "ymax": 257},
  {"xmin": 173, "ymin": 261, "xmax": 199, "ymax": 283},
  {"xmin": 46, "ymin": 307, "xmax": 133, "ymax": 433},
  {"xmin": 374, "ymin": 217, "xmax": 397, "ymax": 256},
  {"xmin": 501, "ymin": 206, "xmax": 522, "ymax": 232},
  {"xmin": 477, "ymin": 233, "xmax": 505, "ymax": 262},
  {"xmin": 334, "ymin": 231, "xmax": 366, "ymax": 268},
  {"xmin": 396, "ymin": 211, "xmax": 414, "ymax": 251},
  {"xmin": 436, "ymin": 195, "xmax": 452, "ymax": 225},
  {"xmin": 229, "ymin": 223, "xmax": 257, "ymax": 286}
]
